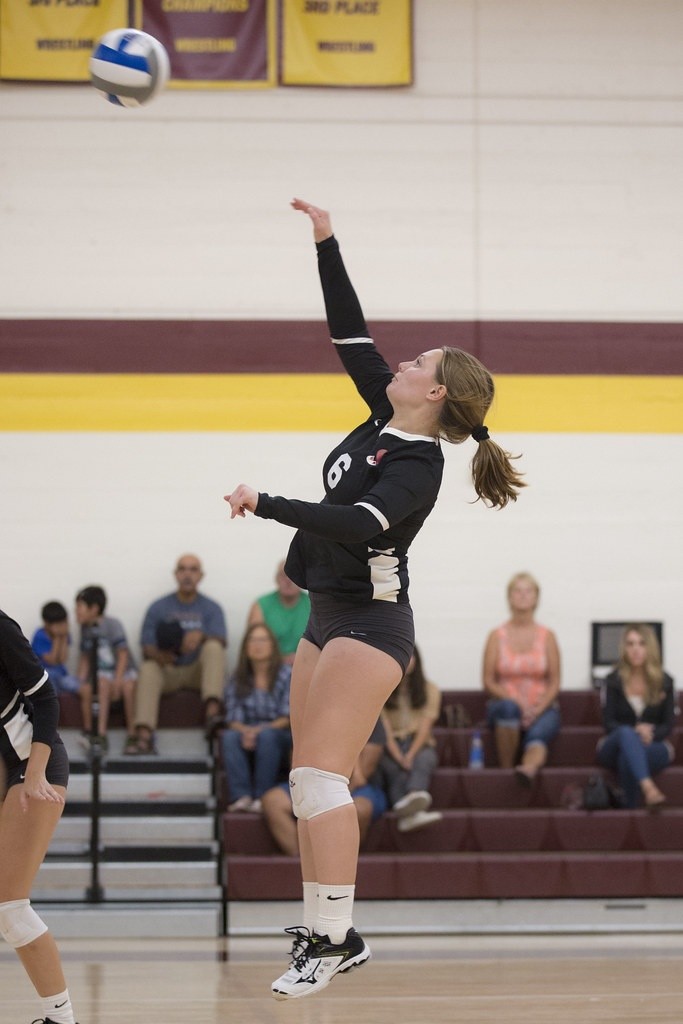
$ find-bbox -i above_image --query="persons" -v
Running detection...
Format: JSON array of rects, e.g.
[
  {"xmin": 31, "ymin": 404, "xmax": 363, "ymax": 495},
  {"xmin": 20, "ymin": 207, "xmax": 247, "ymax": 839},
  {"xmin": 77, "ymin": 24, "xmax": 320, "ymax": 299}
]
[
  {"xmin": 484, "ymin": 572, "xmax": 561, "ymax": 785},
  {"xmin": 223, "ymin": 198, "xmax": 524, "ymax": 1011},
  {"xmin": 75, "ymin": 586, "xmax": 140, "ymax": 757},
  {"xmin": 592, "ymin": 621, "xmax": 676, "ymax": 811},
  {"xmin": 133, "ymin": 555, "xmax": 230, "ymax": 755},
  {"xmin": 261, "ymin": 717, "xmax": 386, "ymax": 856},
  {"xmin": 222, "ymin": 622, "xmax": 292, "ymax": 814},
  {"xmin": 246, "ymin": 560, "xmax": 314, "ymax": 668},
  {"xmin": 378, "ymin": 641, "xmax": 444, "ymax": 830},
  {"xmin": 0, "ymin": 608, "xmax": 84, "ymax": 1024},
  {"xmin": 31, "ymin": 602, "xmax": 96, "ymax": 741}
]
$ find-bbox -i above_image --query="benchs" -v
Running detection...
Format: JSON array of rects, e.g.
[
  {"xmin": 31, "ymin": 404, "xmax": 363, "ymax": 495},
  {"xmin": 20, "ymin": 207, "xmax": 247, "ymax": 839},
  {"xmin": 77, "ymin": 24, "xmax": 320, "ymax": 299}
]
[{"xmin": 51, "ymin": 676, "xmax": 683, "ymax": 897}]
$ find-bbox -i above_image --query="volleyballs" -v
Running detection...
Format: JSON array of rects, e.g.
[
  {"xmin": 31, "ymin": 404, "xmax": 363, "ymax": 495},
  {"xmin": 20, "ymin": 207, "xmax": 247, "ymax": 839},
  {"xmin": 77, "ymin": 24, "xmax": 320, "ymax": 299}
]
[{"xmin": 88, "ymin": 27, "xmax": 172, "ymax": 109}]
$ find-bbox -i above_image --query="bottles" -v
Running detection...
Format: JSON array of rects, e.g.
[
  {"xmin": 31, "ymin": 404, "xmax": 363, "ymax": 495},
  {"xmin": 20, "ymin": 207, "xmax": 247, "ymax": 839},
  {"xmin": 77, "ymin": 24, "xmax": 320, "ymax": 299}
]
[{"xmin": 467, "ymin": 730, "xmax": 485, "ymax": 770}]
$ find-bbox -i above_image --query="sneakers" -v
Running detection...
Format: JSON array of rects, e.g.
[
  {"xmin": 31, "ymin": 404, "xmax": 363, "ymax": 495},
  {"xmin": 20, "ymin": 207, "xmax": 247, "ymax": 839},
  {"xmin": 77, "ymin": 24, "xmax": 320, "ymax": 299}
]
[{"xmin": 271, "ymin": 927, "xmax": 369, "ymax": 999}]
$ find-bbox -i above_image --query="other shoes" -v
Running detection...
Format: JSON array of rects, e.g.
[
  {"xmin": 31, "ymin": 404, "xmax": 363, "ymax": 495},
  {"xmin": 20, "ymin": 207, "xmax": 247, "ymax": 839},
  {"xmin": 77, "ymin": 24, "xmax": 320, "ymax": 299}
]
[
  {"xmin": 88, "ymin": 736, "xmax": 107, "ymax": 759},
  {"xmin": 76, "ymin": 731, "xmax": 92, "ymax": 750},
  {"xmin": 394, "ymin": 791, "xmax": 431, "ymax": 816},
  {"xmin": 246, "ymin": 797, "xmax": 262, "ymax": 813},
  {"xmin": 397, "ymin": 811, "xmax": 440, "ymax": 833},
  {"xmin": 228, "ymin": 795, "xmax": 252, "ymax": 811},
  {"xmin": 515, "ymin": 765, "xmax": 533, "ymax": 789},
  {"xmin": 646, "ymin": 789, "xmax": 666, "ymax": 814}
]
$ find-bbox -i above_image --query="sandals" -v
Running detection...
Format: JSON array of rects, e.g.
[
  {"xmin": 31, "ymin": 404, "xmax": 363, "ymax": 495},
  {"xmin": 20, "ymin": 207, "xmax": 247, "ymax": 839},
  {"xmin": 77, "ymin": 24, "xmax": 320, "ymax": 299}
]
[{"xmin": 124, "ymin": 735, "xmax": 158, "ymax": 756}]
